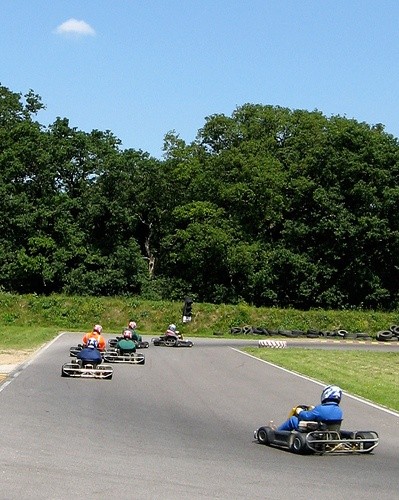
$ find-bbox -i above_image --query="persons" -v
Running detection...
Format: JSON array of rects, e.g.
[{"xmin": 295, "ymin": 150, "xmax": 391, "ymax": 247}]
[
  {"xmin": 77, "ymin": 337, "xmax": 102, "ymax": 369},
  {"xmin": 129, "ymin": 321, "xmax": 142, "ymax": 342},
  {"xmin": 270, "ymin": 385, "xmax": 342, "ymax": 432},
  {"xmin": 165, "ymin": 323, "xmax": 179, "ymax": 339},
  {"xmin": 82, "ymin": 324, "xmax": 105, "ymax": 352},
  {"xmin": 116, "ymin": 329, "xmax": 136, "ymax": 356}
]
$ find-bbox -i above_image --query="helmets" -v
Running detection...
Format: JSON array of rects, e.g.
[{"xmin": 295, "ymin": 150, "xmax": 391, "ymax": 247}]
[
  {"xmin": 87, "ymin": 338, "xmax": 98, "ymax": 348},
  {"xmin": 124, "ymin": 330, "xmax": 132, "ymax": 339},
  {"xmin": 129, "ymin": 321, "xmax": 136, "ymax": 329},
  {"xmin": 169, "ymin": 324, "xmax": 175, "ymax": 331},
  {"xmin": 93, "ymin": 324, "xmax": 102, "ymax": 333},
  {"xmin": 321, "ymin": 384, "xmax": 342, "ymax": 404}
]
